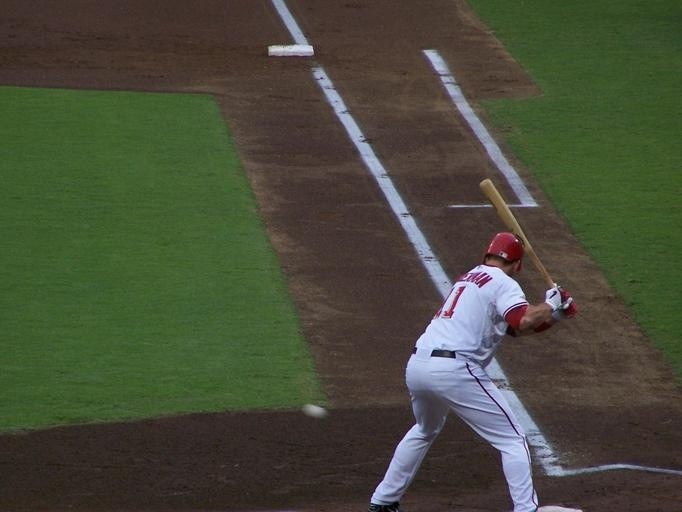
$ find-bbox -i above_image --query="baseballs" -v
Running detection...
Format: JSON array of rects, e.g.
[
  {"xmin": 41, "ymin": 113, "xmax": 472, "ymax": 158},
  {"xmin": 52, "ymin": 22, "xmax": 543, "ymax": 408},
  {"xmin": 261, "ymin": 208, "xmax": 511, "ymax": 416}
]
[{"xmin": 303, "ymin": 404, "xmax": 326, "ymax": 418}]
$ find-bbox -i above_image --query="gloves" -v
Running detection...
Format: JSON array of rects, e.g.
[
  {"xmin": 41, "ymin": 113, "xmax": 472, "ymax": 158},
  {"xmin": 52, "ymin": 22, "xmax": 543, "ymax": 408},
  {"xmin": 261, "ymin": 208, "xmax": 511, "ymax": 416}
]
[{"xmin": 546, "ymin": 286, "xmax": 577, "ymax": 321}]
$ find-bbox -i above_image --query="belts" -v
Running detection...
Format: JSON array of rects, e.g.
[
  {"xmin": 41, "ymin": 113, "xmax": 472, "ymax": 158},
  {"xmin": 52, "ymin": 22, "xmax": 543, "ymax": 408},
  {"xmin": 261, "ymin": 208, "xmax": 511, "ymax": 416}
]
[{"xmin": 412, "ymin": 347, "xmax": 455, "ymax": 358}]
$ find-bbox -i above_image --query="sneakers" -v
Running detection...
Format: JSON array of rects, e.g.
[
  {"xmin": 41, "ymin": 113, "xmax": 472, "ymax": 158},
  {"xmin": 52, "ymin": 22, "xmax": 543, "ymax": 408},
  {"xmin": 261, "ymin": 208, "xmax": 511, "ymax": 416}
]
[{"xmin": 368, "ymin": 502, "xmax": 400, "ymax": 512}]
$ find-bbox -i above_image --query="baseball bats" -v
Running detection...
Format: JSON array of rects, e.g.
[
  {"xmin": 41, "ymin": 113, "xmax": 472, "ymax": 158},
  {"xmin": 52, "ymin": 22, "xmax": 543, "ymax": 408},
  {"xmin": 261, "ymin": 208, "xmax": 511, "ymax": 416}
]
[{"xmin": 480, "ymin": 178, "xmax": 558, "ymax": 288}]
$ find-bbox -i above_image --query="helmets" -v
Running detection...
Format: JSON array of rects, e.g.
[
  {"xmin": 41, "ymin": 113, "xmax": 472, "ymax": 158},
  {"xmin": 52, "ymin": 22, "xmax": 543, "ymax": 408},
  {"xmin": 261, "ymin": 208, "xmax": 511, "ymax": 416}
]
[{"xmin": 485, "ymin": 233, "xmax": 524, "ymax": 262}]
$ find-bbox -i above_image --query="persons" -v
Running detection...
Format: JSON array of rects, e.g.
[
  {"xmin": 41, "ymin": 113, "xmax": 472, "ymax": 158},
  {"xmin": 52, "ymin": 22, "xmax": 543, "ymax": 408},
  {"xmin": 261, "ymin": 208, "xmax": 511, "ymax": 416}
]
[{"xmin": 369, "ymin": 231, "xmax": 578, "ymax": 512}]
